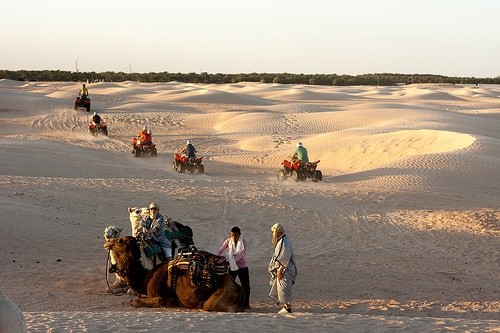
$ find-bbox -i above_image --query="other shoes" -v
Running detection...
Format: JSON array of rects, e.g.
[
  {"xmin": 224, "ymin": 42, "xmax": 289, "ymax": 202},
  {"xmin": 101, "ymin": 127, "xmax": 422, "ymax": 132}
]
[{"xmin": 278, "ymin": 308, "xmax": 291, "ymax": 314}]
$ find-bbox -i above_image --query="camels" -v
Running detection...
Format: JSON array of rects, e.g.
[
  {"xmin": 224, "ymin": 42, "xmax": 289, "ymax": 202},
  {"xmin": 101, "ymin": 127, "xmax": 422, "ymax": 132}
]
[
  {"xmin": 103, "ymin": 226, "xmax": 181, "ymax": 295},
  {"xmin": 128, "ymin": 207, "xmax": 149, "ymax": 237},
  {"xmin": 103, "ymin": 236, "xmax": 246, "ymax": 314}
]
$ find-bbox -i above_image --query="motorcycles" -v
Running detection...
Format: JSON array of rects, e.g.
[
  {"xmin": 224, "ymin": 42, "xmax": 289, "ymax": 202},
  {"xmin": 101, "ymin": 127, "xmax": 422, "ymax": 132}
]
[
  {"xmin": 173, "ymin": 150, "xmax": 204, "ymax": 174},
  {"xmin": 88, "ymin": 121, "xmax": 108, "ymax": 136},
  {"xmin": 74, "ymin": 94, "xmax": 91, "ymax": 112},
  {"xmin": 132, "ymin": 135, "xmax": 158, "ymax": 157},
  {"xmin": 277, "ymin": 156, "xmax": 322, "ymax": 182}
]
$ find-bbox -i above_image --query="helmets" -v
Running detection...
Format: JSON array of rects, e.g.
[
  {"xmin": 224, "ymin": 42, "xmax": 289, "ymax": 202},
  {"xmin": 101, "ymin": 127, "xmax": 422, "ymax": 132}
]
[
  {"xmin": 187, "ymin": 140, "xmax": 192, "ymax": 145},
  {"xmin": 147, "ymin": 129, "xmax": 152, "ymax": 134},
  {"xmin": 143, "ymin": 125, "xmax": 148, "ymax": 130},
  {"xmin": 297, "ymin": 141, "xmax": 303, "ymax": 147},
  {"xmin": 94, "ymin": 112, "xmax": 98, "ymax": 116}
]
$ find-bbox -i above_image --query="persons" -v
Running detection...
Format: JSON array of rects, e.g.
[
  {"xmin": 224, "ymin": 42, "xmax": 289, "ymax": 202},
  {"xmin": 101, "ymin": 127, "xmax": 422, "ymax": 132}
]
[
  {"xmin": 92, "ymin": 111, "xmax": 103, "ymax": 126},
  {"xmin": 78, "ymin": 83, "xmax": 88, "ymax": 96},
  {"xmin": 291, "ymin": 140, "xmax": 309, "ymax": 173},
  {"xmin": 214, "ymin": 226, "xmax": 252, "ymax": 309},
  {"xmin": 268, "ymin": 222, "xmax": 298, "ymax": 313},
  {"xmin": 180, "ymin": 139, "xmax": 198, "ymax": 166},
  {"xmin": 138, "ymin": 201, "xmax": 172, "ymax": 262},
  {"xmin": 137, "ymin": 125, "xmax": 152, "ymax": 151}
]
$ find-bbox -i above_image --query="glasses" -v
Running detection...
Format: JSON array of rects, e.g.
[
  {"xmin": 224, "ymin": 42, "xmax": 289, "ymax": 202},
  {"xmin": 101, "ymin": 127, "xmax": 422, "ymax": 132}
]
[{"xmin": 149, "ymin": 208, "xmax": 159, "ymax": 211}]
